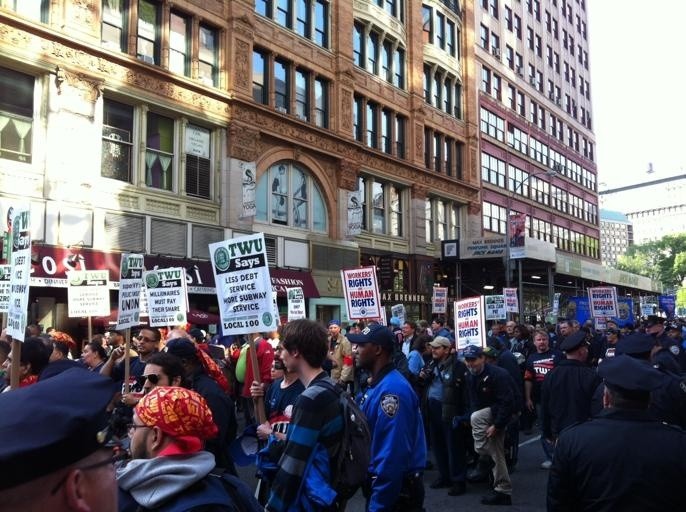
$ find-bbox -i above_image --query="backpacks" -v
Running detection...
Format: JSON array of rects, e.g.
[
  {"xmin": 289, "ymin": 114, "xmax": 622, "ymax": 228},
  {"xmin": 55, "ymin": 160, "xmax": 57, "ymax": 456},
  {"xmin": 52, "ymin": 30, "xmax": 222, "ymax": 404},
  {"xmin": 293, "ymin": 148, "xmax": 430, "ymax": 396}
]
[{"xmin": 321, "ymin": 389, "xmax": 373, "ymax": 503}]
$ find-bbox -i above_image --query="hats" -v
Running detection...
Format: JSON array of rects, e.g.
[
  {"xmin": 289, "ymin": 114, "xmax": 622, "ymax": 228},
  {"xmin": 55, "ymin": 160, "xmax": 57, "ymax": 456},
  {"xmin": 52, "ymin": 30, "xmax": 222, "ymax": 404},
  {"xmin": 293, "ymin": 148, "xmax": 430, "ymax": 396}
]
[
  {"xmin": 596, "ymin": 354, "xmax": 667, "ymax": 399},
  {"xmin": 0, "ymin": 366, "xmax": 123, "ymax": 491},
  {"xmin": 613, "ymin": 331, "xmax": 657, "ymax": 361},
  {"xmin": 606, "ymin": 318, "xmax": 618, "ymax": 325},
  {"xmin": 428, "ymin": 335, "xmax": 451, "ymax": 348},
  {"xmin": 664, "ymin": 325, "xmax": 682, "ymax": 332},
  {"xmin": 345, "ymin": 322, "xmax": 400, "ymax": 353},
  {"xmin": 158, "ymin": 336, "xmax": 199, "ymax": 359},
  {"xmin": 462, "ymin": 344, "xmax": 483, "ymax": 360},
  {"xmin": 104, "ymin": 322, "xmax": 131, "ymax": 336},
  {"xmin": 643, "ymin": 313, "xmax": 666, "ymax": 328},
  {"xmin": 558, "ymin": 330, "xmax": 591, "ymax": 353}
]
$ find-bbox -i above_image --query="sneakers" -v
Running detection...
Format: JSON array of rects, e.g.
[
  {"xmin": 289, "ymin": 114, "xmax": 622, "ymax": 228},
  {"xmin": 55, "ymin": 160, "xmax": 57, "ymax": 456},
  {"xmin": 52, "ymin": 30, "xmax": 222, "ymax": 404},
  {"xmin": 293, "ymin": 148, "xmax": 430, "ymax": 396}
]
[
  {"xmin": 429, "ymin": 475, "xmax": 453, "ymax": 489},
  {"xmin": 539, "ymin": 456, "xmax": 554, "ymax": 472},
  {"xmin": 480, "ymin": 489, "xmax": 514, "ymax": 506},
  {"xmin": 446, "ymin": 482, "xmax": 467, "ymax": 496}
]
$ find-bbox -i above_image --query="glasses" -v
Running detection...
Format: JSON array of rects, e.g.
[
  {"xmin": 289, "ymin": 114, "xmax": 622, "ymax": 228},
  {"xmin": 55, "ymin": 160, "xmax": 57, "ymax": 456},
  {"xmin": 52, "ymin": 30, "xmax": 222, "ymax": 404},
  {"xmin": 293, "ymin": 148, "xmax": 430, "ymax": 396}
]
[
  {"xmin": 49, "ymin": 448, "xmax": 127, "ymax": 496},
  {"xmin": 135, "ymin": 335, "xmax": 159, "ymax": 343},
  {"xmin": 136, "ymin": 373, "xmax": 162, "ymax": 387},
  {"xmin": 123, "ymin": 422, "xmax": 157, "ymax": 437}
]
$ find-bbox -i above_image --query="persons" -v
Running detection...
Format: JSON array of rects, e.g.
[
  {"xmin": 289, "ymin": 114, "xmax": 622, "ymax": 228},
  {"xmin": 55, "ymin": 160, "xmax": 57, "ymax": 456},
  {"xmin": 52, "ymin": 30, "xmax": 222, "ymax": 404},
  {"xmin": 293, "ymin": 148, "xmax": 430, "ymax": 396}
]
[
  {"xmin": 2, "ymin": 315, "xmax": 686, "ymax": 512},
  {"xmin": 293, "ymin": 176, "xmax": 306, "ymax": 227},
  {"xmin": 272, "ymin": 164, "xmax": 285, "ymax": 219}
]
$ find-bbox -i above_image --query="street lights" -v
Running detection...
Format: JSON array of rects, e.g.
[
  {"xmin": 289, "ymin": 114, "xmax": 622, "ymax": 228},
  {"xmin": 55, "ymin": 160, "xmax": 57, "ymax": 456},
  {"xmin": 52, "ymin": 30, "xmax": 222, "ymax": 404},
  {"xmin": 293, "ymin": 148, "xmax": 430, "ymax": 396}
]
[{"xmin": 504, "ymin": 169, "xmax": 557, "ymax": 320}]
